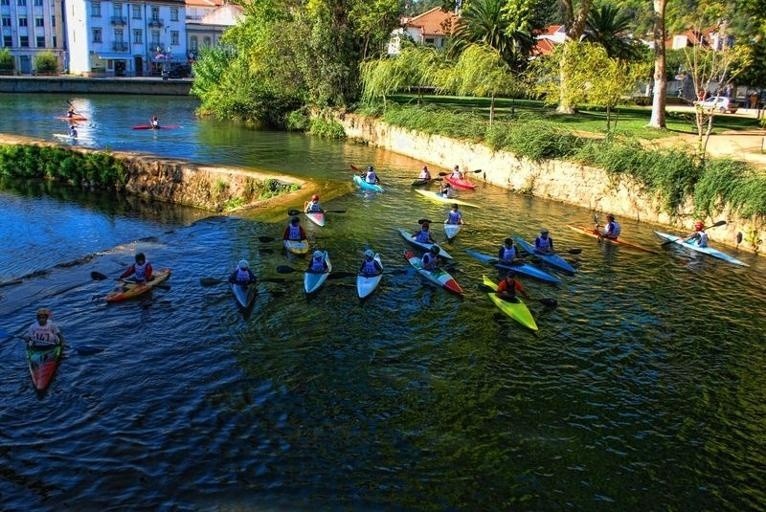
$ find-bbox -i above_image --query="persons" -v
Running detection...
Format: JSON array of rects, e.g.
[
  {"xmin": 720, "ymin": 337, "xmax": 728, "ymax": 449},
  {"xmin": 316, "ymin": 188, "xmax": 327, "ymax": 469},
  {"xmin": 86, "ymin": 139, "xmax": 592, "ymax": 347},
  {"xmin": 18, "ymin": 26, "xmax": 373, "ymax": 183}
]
[
  {"xmin": 534, "ymin": 228, "xmax": 563, "ymax": 255},
  {"xmin": 284, "ymin": 217, "xmax": 306, "ymax": 241},
  {"xmin": 678, "ymin": 221, "xmax": 710, "ymax": 248},
  {"xmin": 67, "ymin": 109, "xmax": 77, "ymax": 118},
  {"xmin": 24, "ymin": 307, "xmax": 63, "ymax": 349},
  {"xmin": 411, "ymin": 222, "xmax": 439, "ymax": 244},
  {"xmin": 359, "ymin": 249, "xmax": 383, "ymax": 275},
  {"xmin": 304, "ymin": 194, "xmax": 327, "ymax": 215},
  {"xmin": 228, "ymin": 259, "xmax": 260, "ymax": 284},
  {"xmin": 308, "ymin": 250, "xmax": 330, "ymax": 273},
  {"xmin": 446, "ymin": 204, "xmax": 465, "ymax": 225},
  {"xmin": 360, "ymin": 166, "xmax": 380, "ymax": 185},
  {"xmin": 495, "ymin": 270, "xmax": 532, "ymax": 301},
  {"xmin": 120, "ymin": 253, "xmax": 152, "ymax": 283},
  {"xmin": 419, "ymin": 166, "xmax": 431, "ymax": 182},
  {"xmin": 421, "ymin": 245, "xmax": 443, "ymax": 271},
  {"xmin": 498, "ymin": 238, "xmax": 522, "ymax": 265},
  {"xmin": 149, "ymin": 115, "xmax": 159, "ymax": 130},
  {"xmin": 594, "ymin": 213, "xmax": 622, "ymax": 239},
  {"xmin": 449, "ymin": 165, "xmax": 464, "ymax": 180}
]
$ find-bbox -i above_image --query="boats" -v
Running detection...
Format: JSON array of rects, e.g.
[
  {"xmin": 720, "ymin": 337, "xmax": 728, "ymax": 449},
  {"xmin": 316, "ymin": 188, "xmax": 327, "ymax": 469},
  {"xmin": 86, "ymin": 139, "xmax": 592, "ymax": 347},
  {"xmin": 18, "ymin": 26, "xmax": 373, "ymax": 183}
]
[
  {"xmin": 285, "ymin": 198, "xmax": 579, "ymax": 332},
  {"xmin": 103, "ymin": 266, "xmax": 170, "ymax": 301},
  {"xmin": 23, "ymin": 328, "xmax": 62, "ymax": 393},
  {"xmin": 350, "ymin": 174, "xmax": 385, "ymax": 193},
  {"xmin": 55, "ymin": 113, "xmax": 86, "ymax": 120},
  {"xmin": 227, "ymin": 269, "xmax": 256, "ymax": 309},
  {"xmin": 130, "ymin": 124, "xmax": 183, "ymax": 130},
  {"xmin": 566, "ymin": 223, "xmax": 658, "ymax": 255},
  {"xmin": 51, "ymin": 132, "xmax": 93, "ymax": 141},
  {"xmin": 653, "ymin": 230, "xmax": 752, "ymax": 269},
  {"xmin": 445, "ymin": 174, "xmax": 478, "ymax": 188},
  {"xmin": 414, "ymin": 187, "xmax": 481, "ymax": 209},
  {"xmin": 410, "ymin": 177, "xmax": 432, "ymax": 186}
]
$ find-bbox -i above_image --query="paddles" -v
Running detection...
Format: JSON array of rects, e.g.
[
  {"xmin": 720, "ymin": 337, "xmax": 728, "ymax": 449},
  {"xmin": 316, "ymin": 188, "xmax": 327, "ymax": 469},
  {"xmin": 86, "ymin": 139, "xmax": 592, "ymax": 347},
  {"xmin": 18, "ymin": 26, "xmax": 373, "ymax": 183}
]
[
  {"xmin": 65, "ymin": 99, "xmax": 84, "ymax": 118},
  {"xmin": 288, "ymin": 210, "xmax": 346, "ymax": 216},
  {"xmin": 276, "ymin": 265, "xmax": 356, "ymax": 279},
  {"xmin": 439, "ymin": 170, "xmax": 481, "ymax": 176},
  {"xmin": 518, "ymin": 248, "xmax": 583, "ymax": 255},
  {"xmin": 418, "ymin": 219, "xmax": 472, "ymax": 226},
  {"xmin": 661, "ymin": 220, "xmax": 726, "ymax": 246},
  {"xmin": 200, "ymin": 277, "xmax": 285, "ymax": 287},
  {"xmin": 350, "ymin": 165, "xmax": 382, "ymax": 183},
  {"xmin": 399, "ymin": 176, "xmax": 444, "ymax": 181},
  {"xmin": 258, "ymin": 236, "xmax": 333, "ymax": 242},
  {"xmin": 356, "ymin": 269, "xmax": 409, "ymax": 275},
  {"xmin": 488, "ymin": 257, "xmax": 540, "ymax": 263},
  {"xmin": 91, "ymin": 271, "xmax": 171, "ymax": 291},
  {"xmin": 411, "ymin": 229, "xmax": 454, "ymax": 251},
  {"xmin": 479, "ymin": 283, "xmax": 557, "ymax": 307},
  {"xmin": 0, "ymin": 329, "xmax": 104, "ymax": 356}
]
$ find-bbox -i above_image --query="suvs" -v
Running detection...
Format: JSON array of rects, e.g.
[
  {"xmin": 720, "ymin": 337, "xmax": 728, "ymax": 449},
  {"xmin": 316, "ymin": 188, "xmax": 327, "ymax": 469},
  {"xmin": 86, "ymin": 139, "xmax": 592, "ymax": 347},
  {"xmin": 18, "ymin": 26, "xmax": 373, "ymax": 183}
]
[{"xmin": 697, "ymin": 95, "xmax": 738, "ymax": 113}]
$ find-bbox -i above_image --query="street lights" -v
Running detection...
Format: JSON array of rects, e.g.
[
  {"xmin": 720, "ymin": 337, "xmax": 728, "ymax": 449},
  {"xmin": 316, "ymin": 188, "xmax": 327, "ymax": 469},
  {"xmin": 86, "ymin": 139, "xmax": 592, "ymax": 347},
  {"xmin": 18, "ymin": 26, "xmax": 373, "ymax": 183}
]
[{"xmin": 155, "ymin": 44, "xmax": 172, "ymax": 71}]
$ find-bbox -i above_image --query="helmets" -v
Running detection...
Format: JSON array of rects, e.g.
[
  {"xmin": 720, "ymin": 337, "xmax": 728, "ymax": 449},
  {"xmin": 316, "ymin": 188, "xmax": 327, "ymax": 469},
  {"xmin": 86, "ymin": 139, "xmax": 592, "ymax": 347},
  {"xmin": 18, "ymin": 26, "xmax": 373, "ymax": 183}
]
[
  {"xmin": 314, "ymin": 251, "xmax": 323, "ymax": 259},
  {"xmin": 135, "ymin": 252, "xmax": 146, "ymax": 264},
  {"xmin": 422, "ymin": 222, "xmax": 429, "ymax": 230},
  {"xmin": 539, "ymin": 228, "xmax": 550, "ymax": 235},
  {"xmin": 454, "ymin": 165, "xmax": 459, "ymax": 170},
  {"xmin": 453, "ymin": 203, "xmax": 458, "ymax": 209},
  {"xmin": 368, "ymin": 166, "xmax": 374, "ymax": 171},
  {"xmin": 36, "ymin": 309, "xmax": 51, "ymax": 321},
  {"xmin": 238, "ymin": 259, "xmax": 250, "ymax": 271},
  {"xmin": 504, "ymin": 238, "xmax": 513, "ymax": 246},
  {"xmin": 505, "ymin": 270, "xmax": 516, "ymax": 280},
  {"xmin": 311, "ymin": 194, "xmax": 319, "ymax": 202},
  {"xmin": 365, "ymin": 250, "xmax": 375, "ymax": 261},
  {"xmin": 291, "ymin": 216, "xmax": 299, "ymax": 225},
  {"xmin": 430, "ymin": 244, "xmax": 440, "ymax": 256},
  {"xmin": 152, "ymin": 116, "xmax": 157, "ymax": 120},
  {"xmin": 606, "ymin": 213, "xmax": 615, "ymax": 220},
  {"xmin": 695, "ymin": 222, "xmax": 705, "ymax": 228},
  {"xmin": 422, "ymin": 165, "xmax": 427, "ymax": 170}
]
